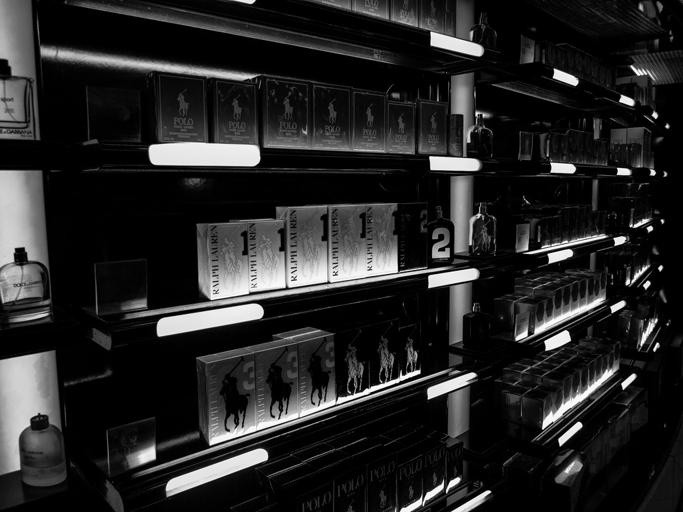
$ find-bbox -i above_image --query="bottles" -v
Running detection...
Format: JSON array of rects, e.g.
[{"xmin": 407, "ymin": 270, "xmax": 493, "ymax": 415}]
[{"xmin": 18, "ymin": 414, "xmax": 66, "ymax": 486}]
